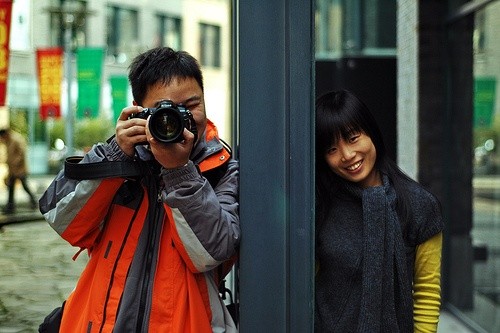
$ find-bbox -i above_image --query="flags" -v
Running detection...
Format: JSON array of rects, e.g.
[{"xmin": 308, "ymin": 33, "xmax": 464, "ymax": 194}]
[
  {"xmin": 34, "ymin": 47, "xmax": 62, "ymax": 119},
  {"xmin": 75, "ymin": 46, "xmax": 107, "ymax": 118},
  {"xmin": 1, "ymin": 0, "xmax": 14, "ymax": 106},
  {"xmin": 109, "ymin": 75, "xmax": 128, "ymax": 126}
]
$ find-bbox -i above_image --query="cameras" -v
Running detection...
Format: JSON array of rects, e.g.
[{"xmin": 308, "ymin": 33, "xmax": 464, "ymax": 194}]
[{"xmin": 129, "ymin": 99, "xmax": 199, "ymax": 152}]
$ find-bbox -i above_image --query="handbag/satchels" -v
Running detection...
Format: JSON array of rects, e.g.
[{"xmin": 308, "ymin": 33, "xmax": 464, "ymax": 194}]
[{"xmin": 39, "ymin": 307, "xmax": 63, "ymax": 333}]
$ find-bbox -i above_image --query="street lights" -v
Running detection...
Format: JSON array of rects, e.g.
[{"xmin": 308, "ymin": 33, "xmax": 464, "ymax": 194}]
[{"xmin": 46, "ymin": 2, "xmax": 95, "ymax": 159}]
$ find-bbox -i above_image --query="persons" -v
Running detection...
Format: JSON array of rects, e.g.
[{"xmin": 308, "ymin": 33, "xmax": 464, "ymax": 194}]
[
  {"xmin": 40, "ymin": 48, "xmax": 241, "ymax": 333},
  {"xmin": 0, "ymin": 129, "xmax": 37, "ymax": 213},
  {"xmin": 314, "ymin": 88, "xmax": 443, "ymax": 333}
]
